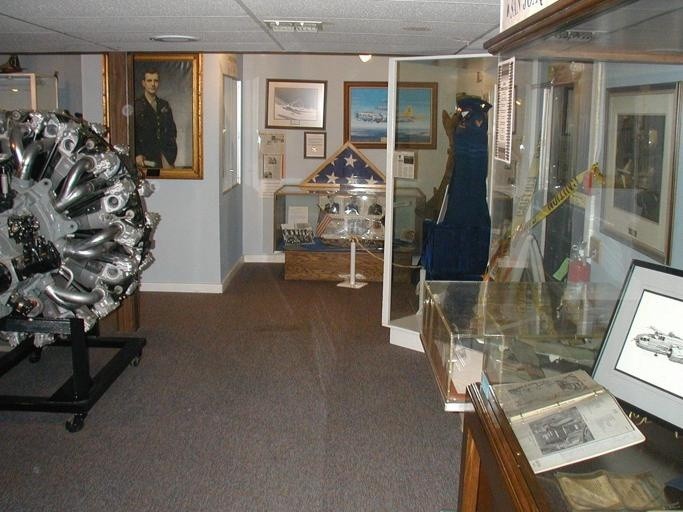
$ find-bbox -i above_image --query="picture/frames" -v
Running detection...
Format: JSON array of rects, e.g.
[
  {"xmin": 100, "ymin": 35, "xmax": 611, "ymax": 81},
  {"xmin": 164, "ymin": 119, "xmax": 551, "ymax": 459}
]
[
  {"xmin": 344, "ymin": 81, "xmax": 437, "ymax": 149},
  {"xmin": 133, "ymin": 54, "xmax": 203, "ymax": 179},
  {"xmin": 590, "ymin": 259, "xmax": 683, "ymax": 436},
  {"xmin": 598, "ymin": 81, "xmax": 683, "ymax": 266},
  {"xmin": 265, "ymin": 79, "xmax": 327, "ymax": 158}
]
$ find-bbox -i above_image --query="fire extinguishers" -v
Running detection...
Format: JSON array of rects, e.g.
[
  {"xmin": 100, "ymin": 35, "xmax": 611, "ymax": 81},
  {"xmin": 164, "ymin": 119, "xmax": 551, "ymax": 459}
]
[{"xmin": 563, "ymin": 244, "xmax": 592, "ymax": 301}]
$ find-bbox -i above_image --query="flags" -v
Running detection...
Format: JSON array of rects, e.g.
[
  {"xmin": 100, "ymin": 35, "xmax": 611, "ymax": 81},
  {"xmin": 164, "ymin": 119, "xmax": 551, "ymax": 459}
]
[{"xmin": 313, "ymin": 208, "xmax": 333, "ymax": 239}]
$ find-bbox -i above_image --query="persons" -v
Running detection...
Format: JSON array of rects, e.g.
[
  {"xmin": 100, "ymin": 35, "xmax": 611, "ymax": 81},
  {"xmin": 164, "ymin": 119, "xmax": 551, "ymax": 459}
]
[{"xmin": 133, "ymin": 67, "xmax": 177, "ymax": 176}]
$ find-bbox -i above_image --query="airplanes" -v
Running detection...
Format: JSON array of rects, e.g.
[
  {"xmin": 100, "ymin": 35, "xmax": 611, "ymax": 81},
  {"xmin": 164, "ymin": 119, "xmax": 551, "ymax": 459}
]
[
  {"xmin": 356, "ymin": 105, "xmax": 426, "ymax": 123},
  {"xmin": 632, "ymin": 325, "xmax": 683, "ymax": 364}
]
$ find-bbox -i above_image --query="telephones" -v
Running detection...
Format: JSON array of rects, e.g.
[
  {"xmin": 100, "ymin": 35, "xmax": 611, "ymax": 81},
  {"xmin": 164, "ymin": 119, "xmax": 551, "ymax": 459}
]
[{"xmin": 394, "ymin": 151, "xmax": 415, "ymax": 180}]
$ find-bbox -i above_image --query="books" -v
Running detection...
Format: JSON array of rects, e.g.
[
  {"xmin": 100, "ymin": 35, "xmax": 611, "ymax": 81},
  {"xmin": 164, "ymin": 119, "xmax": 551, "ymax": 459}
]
[{"xmin": 488, "ymin": 366, "xmax": 650, "ymax": 476}]
